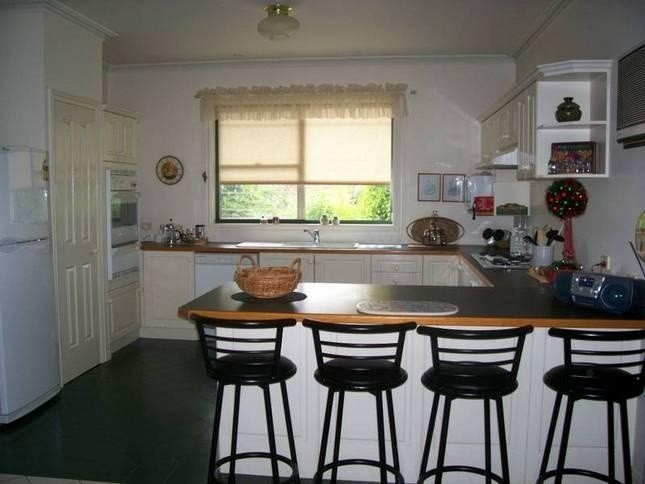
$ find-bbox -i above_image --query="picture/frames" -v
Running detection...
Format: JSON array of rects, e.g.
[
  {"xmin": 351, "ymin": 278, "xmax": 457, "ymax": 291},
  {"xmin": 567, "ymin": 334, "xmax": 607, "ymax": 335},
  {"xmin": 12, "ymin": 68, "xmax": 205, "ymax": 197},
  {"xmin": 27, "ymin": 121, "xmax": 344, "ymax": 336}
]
[
  {"xmin": 442, "ymin": 173, "xmax": 465, "ymax": 202},
  {"xmin": 418, "ymin": 173, "xmax": 441, "ymax": 202}
]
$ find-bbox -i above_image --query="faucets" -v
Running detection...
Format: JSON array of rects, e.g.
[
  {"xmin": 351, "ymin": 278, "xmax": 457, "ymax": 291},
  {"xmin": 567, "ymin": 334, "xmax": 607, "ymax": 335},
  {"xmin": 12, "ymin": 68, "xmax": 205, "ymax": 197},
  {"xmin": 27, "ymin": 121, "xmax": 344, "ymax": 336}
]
[{"xmin": 303, "ymin": 229, "xmax": 321, "ymax": 245}]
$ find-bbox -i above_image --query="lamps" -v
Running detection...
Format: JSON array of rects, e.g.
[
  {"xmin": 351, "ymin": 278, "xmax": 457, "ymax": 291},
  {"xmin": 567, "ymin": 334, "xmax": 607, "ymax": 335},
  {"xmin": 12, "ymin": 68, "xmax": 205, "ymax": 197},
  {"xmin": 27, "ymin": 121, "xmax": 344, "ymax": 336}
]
[{"xmin": 257, "ymin": 1, "xmax": 300, "ymax": 41}]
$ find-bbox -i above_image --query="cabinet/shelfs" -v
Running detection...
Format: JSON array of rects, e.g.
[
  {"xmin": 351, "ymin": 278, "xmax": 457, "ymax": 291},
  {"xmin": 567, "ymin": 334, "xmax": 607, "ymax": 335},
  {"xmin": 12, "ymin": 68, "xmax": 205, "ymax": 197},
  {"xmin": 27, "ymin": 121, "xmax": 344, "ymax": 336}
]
[
  {"xmin": 476, "ymin": 59, "xmax": 613, "ymax": 180},
  {"xmin": 102, "ymin": 104, "xmax": 140, "ymax": 165}
]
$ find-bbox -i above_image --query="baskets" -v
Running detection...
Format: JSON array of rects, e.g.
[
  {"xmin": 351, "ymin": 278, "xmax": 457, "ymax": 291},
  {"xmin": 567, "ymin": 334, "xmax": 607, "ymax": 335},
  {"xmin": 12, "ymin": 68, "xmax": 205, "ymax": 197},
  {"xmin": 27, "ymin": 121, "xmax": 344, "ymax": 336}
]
[{"xmin": 233, "ymin": 255, "xmax": 302, "ymax": 300}]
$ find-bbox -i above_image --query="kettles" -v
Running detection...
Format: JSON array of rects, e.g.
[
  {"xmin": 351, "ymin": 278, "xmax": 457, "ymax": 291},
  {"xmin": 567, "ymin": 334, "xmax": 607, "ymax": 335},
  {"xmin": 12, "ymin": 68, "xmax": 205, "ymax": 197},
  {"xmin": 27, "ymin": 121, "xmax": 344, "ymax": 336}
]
[{"xmin": 423, "ymin": 222, "xmax": 447, "ymax": 246}]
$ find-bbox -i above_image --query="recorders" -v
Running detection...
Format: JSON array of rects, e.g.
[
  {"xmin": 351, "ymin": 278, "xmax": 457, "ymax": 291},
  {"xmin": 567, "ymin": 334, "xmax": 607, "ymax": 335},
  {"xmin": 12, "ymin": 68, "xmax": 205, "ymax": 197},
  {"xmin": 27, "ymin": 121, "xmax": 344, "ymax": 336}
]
[{"xmin": 553, "ymin": 269, "xmax": 639, "ymax": 314}]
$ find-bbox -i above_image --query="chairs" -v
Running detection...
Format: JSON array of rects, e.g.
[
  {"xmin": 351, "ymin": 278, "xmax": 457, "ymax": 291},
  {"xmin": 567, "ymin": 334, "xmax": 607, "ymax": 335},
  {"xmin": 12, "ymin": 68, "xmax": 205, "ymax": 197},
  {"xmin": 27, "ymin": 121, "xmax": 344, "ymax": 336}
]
[
  {"xmin": 189, "ymin": 313, "xmax": 301, "ymax": 484},
  {"xmin": 302, "ymin": 318, "xmax": 417, "ymax": 484},
  {"xmin": 535, "ymin": 325, "xmax": 645, "ymax": 483},
  {"xmin": 416, "ymin": 325, "xmax": 534, "ymax": 484}
]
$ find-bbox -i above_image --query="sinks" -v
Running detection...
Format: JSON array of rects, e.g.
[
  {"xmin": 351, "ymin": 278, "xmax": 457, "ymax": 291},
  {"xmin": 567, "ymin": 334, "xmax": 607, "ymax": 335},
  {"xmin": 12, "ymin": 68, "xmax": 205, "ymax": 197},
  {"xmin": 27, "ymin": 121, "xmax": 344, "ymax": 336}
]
[{"xmin": 281, "ymin": 242, "xmax": 359, "ymax": 249}]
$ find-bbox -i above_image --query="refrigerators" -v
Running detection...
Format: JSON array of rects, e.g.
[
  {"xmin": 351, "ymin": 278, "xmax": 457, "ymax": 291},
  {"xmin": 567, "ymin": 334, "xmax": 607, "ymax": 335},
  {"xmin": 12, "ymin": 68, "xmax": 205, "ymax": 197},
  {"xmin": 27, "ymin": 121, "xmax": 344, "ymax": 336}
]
[{"xmin": 0, "ymin": 142, "xmax": 63, "ymax": 423}]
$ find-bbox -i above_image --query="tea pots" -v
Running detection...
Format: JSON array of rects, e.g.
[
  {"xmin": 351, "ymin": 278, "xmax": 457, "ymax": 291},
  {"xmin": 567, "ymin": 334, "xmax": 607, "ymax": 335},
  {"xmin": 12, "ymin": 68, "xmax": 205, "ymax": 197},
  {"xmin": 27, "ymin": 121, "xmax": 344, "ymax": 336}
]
[{"xmin": 160, "ymin": 224, "xmax": 181, "ymax": 250}]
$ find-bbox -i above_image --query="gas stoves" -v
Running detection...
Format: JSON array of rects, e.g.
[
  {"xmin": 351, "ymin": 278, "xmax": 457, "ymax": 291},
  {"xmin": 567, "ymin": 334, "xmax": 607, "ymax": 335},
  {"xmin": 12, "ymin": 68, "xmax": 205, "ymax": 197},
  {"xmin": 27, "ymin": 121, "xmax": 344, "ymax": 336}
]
[{"xmin": 471, "ymin": 250, "xmax": 532, "ymax": 269}]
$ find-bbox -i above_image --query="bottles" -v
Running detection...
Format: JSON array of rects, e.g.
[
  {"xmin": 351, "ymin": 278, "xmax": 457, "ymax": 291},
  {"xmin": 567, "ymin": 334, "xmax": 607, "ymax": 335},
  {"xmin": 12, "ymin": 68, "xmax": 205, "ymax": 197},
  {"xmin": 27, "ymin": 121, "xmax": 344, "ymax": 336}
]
[
  {"xmin": 320, "ymin": 215, "xmax": 329, "ymax": 225},
  {"xmin": 261, "ymin": 216, "xmax": 268, "ymax": 224},
  {"xmin": 332, "ymin": 216, "xmax": 339, "ymax": 225},
  {"xmin": 195, "ymin": 224, "xmax": 204, "ymax": 239},
  {"xmin": 272, "ymin": 217, "xmax": 279, "ymax": 225}
]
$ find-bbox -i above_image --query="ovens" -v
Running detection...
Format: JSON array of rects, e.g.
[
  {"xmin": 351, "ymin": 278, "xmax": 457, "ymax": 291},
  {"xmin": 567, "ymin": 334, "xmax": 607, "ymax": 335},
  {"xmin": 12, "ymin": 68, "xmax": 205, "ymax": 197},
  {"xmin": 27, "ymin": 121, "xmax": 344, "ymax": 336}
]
[{"xmin": 104, "ymin": 168, "xmax": 142, "ymax": 280}]
